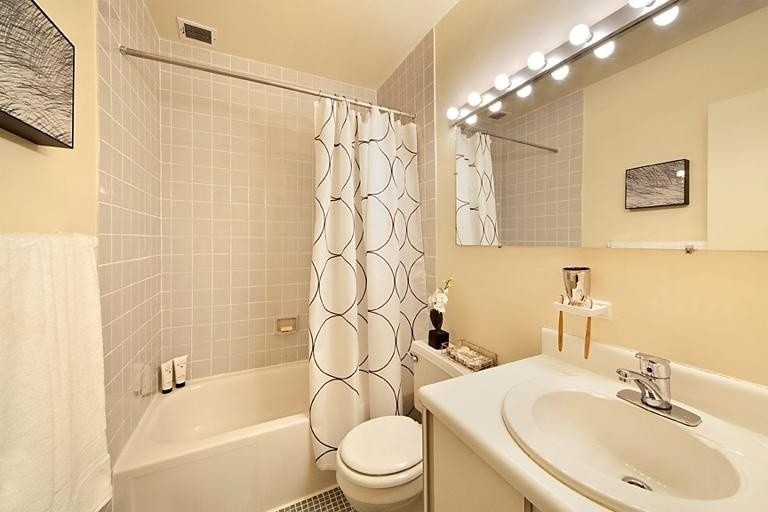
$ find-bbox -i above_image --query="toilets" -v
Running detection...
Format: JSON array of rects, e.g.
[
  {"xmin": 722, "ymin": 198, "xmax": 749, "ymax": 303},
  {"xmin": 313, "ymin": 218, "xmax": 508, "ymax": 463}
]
[{"xmin": 335, "ymin": 338, "xmax": 476, "ymax": 512}]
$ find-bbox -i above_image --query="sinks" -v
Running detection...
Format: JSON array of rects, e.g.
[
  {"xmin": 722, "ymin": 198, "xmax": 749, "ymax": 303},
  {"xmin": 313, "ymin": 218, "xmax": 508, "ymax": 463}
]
[{"xmin": 502, "ymin": 373, "xmax": 768, "ymax": 511}]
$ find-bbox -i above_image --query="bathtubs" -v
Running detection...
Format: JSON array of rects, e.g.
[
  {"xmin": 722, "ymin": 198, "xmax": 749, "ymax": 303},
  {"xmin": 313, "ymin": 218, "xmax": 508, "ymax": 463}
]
[{"xmin": 111, "ymin": 357, "xmax": 340, "ymax": 511}]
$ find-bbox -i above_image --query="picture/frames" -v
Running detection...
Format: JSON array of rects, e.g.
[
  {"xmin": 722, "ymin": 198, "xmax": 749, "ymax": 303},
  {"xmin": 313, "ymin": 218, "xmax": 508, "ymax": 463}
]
[
  {"xmin": 622, "ymin": 158, "xmax": 690, "ymax": 210},
  {"xmin": 0, "ymin": 1, "xmax": 76, "ymax": 150}
]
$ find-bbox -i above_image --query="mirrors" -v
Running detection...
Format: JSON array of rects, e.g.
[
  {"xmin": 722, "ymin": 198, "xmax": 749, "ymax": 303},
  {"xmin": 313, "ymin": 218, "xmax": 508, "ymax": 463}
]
[{"xmin": 447, "ymin": 0, "xmax": 766, "ymax": 249}]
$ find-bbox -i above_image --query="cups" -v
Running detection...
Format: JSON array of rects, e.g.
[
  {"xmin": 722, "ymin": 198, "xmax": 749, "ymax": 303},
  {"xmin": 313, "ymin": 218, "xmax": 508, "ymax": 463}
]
[{"xmin": 562, "ymin": 267, "xmax": 590, "ymax": 305}]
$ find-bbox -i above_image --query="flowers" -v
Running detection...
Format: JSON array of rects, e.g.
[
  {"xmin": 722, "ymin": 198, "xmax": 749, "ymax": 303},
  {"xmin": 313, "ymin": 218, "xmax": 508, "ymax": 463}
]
[{"xmin": 424, "ymin": 275, "xmax": 457, "ymax": 311}]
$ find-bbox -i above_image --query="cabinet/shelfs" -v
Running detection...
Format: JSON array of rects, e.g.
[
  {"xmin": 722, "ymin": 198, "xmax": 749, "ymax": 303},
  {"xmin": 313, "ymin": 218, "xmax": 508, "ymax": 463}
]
[{"xmin": 424, "ymin": 412, "xmax": 534, "ymax": 512}]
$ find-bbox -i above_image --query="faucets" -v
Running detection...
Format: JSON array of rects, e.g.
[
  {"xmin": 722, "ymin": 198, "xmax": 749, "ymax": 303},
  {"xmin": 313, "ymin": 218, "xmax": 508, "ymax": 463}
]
[{"xmin": 616, "ymin": 352, "xmax": 672, "ymax": 409}]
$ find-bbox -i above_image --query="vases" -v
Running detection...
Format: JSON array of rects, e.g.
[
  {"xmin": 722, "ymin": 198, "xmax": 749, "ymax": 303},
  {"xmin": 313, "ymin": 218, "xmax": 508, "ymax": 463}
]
[{"xmin": 426, "ymin": 309, "xmax": 451, "ymax": 349}]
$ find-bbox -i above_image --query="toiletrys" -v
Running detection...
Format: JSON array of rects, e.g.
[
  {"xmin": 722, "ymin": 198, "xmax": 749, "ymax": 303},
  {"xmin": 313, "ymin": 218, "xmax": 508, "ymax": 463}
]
[{"xmin": 161, "ymin": 355, "xmax": 188, "ymax": 394}]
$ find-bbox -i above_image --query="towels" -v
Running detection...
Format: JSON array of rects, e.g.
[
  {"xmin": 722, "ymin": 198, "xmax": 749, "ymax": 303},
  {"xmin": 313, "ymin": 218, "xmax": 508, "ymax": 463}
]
[{"xmin": 0, "ymin": 228, "xmax": 114, "ymax": 512}]
